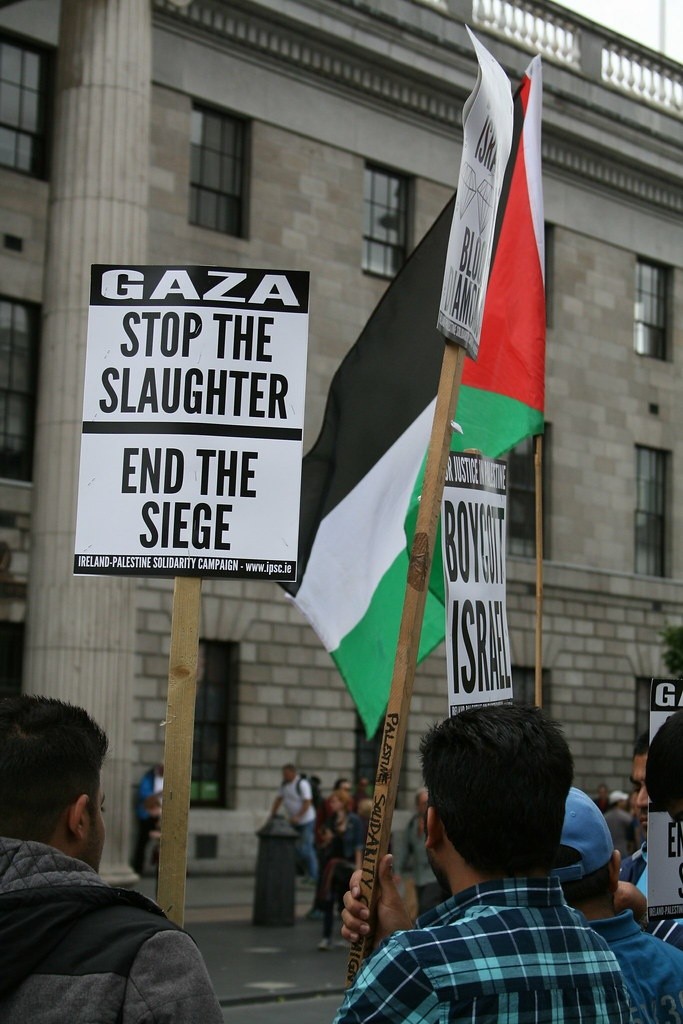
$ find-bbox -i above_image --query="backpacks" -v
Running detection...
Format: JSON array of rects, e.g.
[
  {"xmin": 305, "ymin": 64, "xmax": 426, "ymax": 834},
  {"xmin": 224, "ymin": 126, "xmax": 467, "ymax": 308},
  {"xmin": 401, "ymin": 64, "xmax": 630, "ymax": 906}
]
[{"xmin": 283, "ymin": 778, "xmax": 318, "ymax": 808}]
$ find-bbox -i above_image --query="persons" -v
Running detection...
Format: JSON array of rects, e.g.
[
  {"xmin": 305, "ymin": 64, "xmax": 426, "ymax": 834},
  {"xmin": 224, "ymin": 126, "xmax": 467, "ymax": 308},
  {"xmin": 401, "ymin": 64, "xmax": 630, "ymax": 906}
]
[
  {"xmin": 132, "ymin": 705, "xmax": 683, "ymax": 1024},
  {"xmin": 0, "ymin": 691, "xmax": 225, "ymax": 1024}
]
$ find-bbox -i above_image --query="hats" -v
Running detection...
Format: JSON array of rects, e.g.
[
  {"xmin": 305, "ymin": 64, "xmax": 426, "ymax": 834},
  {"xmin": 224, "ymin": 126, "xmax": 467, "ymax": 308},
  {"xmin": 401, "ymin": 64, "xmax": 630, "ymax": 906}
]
[
  {"xmin": 607, "ymin": 790, "xmax": 629, "ymax": 803},
  {"xmin": 549, "ymin": 788, "xmax": 613, "ymax": 884}
]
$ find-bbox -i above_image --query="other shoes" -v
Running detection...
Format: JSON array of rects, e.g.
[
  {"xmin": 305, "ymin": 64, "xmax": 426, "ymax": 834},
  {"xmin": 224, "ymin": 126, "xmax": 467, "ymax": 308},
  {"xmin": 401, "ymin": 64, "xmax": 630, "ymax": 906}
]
[{"xmin": 318, "ymin": 938, "xmax": 331, "ymax": 950}]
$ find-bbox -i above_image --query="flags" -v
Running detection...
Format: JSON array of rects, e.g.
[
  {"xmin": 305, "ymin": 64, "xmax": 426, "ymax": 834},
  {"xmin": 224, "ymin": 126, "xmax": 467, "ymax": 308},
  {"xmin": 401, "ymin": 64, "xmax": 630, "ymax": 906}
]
[{"xmin": 276, "ymin": 57, "xmax": 544, "ymax": 740}]
